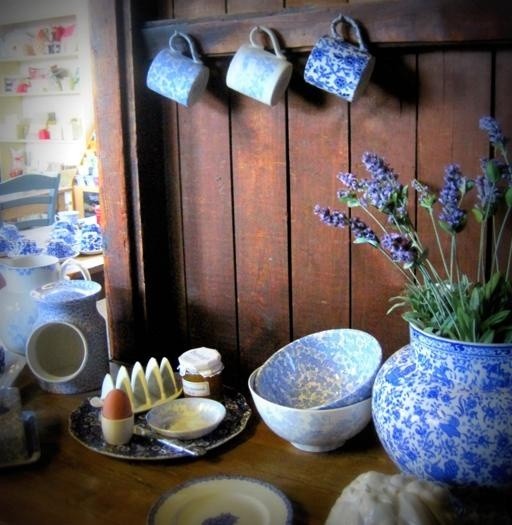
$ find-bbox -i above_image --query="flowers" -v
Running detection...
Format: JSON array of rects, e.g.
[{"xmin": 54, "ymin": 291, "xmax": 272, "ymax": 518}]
[
  {"xmin": 32, "ymin": 24, "xmax": 75, "ymax": 55},
  {"xmin": 314, "ymin": 116, "xmax": 512, "ymax": 343}
]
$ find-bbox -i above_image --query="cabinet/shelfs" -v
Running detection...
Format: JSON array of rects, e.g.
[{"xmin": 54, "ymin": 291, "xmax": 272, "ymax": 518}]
[{"xmin": 0, "ymin": 14, "xmax": 84, "ymax": 181}]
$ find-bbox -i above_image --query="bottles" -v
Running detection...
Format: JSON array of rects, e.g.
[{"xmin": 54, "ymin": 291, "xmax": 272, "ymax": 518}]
[
  {"xmin": 8, "ymin": 146, "xmax": 27, "ymax": 179},
  {"xmin": 179, "ymin": 347, "xmax": 224, "ymax": 398},
  {"xmin": 69, "ymin": 118, "xmax": 78, "ymax": 139},
  {"xmin": 44, "ymin": 112, "xmax": 59, "ymax": 139}
]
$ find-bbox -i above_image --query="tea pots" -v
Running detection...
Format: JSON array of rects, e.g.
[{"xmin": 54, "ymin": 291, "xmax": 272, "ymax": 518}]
[{"xmin": 0, "ymin": 257, "xmax": 93, "ymax": 357}]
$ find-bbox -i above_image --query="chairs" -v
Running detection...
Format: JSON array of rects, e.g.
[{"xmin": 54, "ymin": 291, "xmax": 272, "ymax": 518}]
[
  {"xmin": 0, "ymin": 173, "xmax": 60, "ymax": 230},
  {"xmin": 16, "ymin": 187, "xmax": 84, "ymax": 228},
  {"xmin": 59, "ymin": 168, "xmax": 77, "ymax": 188}
]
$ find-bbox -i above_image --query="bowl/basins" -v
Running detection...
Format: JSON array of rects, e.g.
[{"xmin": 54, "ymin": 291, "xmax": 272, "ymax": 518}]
[{"xmin": 247, "ymin": 327, "xmax": 383, "ymax": 453}]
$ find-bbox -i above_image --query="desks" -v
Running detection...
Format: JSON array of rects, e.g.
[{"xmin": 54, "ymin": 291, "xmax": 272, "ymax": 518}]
[{"xmin": 0, "ymin": 216, "xmax": 103, "ymax": 280}]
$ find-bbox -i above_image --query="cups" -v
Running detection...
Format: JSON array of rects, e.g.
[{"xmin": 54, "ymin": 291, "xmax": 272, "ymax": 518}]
[
  {"xmin": 98, "ymin": 409, "xmax": 134, "ymax": 446},
  {"xmin": 145, "ymin": 29, "xmax": 213, "ymax": 107},
  {"xmin": 14, "ymin": 122, "xmax": 31, "ymax": 141},
  {"xmin": 54, "ymin": 210, "xmax": 79, "ymax": 223},
  {"xmin": 3, "ymin": 75, "xmax": 16, "ymax": 96},
  {"xmin": 302, "ymin": 17, "xmax": 376, "ymax": 104},
  {"xmin": 221, "ymin": 25, "xmax": 297, "ymax": 105},
  {"xmin": 95, "ymin": 205, "xmax": 100, "ymax": 222}
]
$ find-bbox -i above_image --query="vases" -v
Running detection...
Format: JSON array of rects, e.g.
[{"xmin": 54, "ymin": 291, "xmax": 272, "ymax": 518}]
[
  {"xmin": 42, "ymin": 40, "xmax": 61, "ymax": 55},
  {"xmin": 371, "ymin": 322, "xmax": 512, "ymax": 488}
]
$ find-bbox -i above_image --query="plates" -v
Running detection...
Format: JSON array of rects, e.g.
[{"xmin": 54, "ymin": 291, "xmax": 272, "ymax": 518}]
[
  {"xmin": 145, "ymin": 396, "xmax": 228, "ymax": 440},
  {"xmin": 69, "ymin": 378, "xmax": 253, "ymax": 462},
  {"xmin": 149, "ymin": 473, "xmax": 296, "ymax": 525},
  {"xmin": 1, "ymin": 222, "xmax": 103, "ymax": 262}
]
